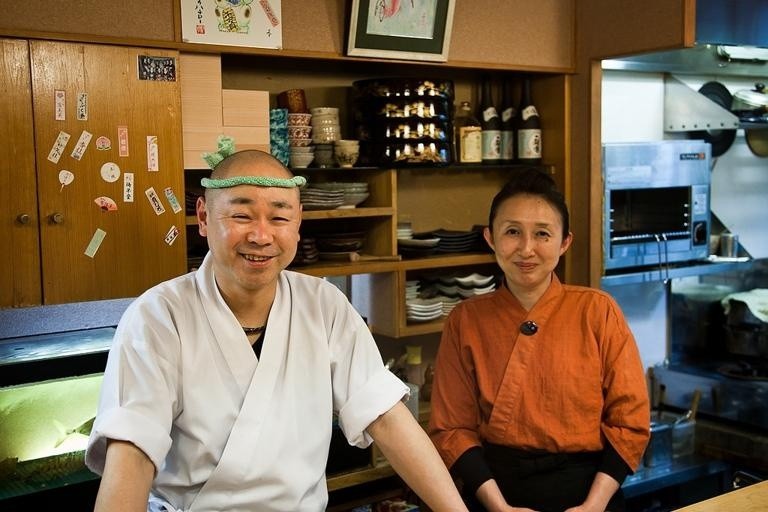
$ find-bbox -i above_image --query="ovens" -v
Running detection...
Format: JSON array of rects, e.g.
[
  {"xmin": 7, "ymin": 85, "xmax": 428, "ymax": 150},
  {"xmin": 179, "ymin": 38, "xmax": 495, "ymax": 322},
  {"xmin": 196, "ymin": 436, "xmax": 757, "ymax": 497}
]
[{"xmin": 599, "ymin": 142, "xmax": 712, "ymax": 275}]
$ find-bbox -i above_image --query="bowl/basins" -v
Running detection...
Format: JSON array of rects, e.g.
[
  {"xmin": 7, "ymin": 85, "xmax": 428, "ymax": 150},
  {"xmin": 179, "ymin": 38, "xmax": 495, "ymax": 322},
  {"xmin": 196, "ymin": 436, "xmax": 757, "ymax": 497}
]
[{"xmin": 269, "ymin": 89, "xmax": 358, "ymax": 170}]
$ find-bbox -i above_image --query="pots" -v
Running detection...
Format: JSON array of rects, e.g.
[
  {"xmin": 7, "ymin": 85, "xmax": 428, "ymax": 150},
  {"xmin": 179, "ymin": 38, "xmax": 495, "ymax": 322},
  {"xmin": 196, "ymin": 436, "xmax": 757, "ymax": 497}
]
[
  {"xmin": 730, "ymin": 84, "xmax": 768, "ymax": 118},
  {"xmin": 723, "ymin": 293, "xmax": 768, "ymax": 359}
]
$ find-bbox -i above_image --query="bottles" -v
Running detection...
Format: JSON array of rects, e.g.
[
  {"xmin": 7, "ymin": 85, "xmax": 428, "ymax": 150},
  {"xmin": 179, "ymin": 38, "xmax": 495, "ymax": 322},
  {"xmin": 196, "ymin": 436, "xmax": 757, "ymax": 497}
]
[{"xmin": 452, "ymin": 74, "xmax": 545, "ymax": 166}]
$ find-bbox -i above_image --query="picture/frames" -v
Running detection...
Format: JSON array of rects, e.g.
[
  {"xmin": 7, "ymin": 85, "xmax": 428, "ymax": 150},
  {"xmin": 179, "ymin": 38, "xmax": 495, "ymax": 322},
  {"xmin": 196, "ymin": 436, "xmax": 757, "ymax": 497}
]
[{"xmin": 346, "ymin": 0, "xmax": 456, "ymax": 63}]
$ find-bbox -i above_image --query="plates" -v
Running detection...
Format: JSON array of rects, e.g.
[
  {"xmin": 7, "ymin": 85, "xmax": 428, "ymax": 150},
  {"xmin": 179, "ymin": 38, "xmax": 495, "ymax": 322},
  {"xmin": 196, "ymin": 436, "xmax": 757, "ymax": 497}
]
[
  {"xmin": 183, "ymin": 187, "xmax": 199, "ymax": 211},
  {"xmin": 300, "ymin": 219, "xmax": 497, "ymax": 320},
  {"xmin": 183, "ymin": 253, "xmax": 204, "ymax": 268},
  {"xmin": 296, "ymin": 180, "xmax": 367, "ymax": 210}
]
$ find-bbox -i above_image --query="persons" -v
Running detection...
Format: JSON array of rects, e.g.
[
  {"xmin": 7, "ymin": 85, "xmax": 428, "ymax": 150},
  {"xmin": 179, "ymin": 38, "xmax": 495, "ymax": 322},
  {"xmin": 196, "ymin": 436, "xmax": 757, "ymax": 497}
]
[
  {"xmin": 430, "ymin": 161, "xmax": 653, "ymax": 512},
  {"xmin": 88, "ymin": 146, "xmax": 474, "ymax": 512}
]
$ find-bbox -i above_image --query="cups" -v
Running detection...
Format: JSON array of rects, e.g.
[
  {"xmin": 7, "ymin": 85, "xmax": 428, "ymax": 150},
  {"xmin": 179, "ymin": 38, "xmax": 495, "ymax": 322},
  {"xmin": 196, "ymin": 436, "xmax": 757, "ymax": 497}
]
[{"xmin": 717, "ymin": 232, "xmax": 739, "ymax": 259}]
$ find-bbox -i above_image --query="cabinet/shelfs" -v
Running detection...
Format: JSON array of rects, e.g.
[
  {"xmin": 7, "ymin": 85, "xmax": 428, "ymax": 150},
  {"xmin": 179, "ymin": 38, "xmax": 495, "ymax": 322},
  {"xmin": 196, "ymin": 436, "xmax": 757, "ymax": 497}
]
[
  {"xmin": 183, "ymin": 163, "xmax": 558, "ymax": 493},
  {"xmin": 0, "ymin": 36, "xmax": 188, "ymax": 309}
]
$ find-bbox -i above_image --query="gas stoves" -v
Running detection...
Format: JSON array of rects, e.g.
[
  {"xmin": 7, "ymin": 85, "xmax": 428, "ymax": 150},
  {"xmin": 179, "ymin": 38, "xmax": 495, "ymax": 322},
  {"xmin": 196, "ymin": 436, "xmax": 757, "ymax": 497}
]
[{"xmin": 652, "ymin": 364, "xmax": 768, "ymax": 430}]
